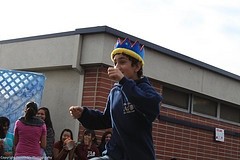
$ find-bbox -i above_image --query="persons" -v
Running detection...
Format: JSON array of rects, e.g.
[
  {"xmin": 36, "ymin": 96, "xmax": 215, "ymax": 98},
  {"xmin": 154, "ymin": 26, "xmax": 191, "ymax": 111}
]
[
  {"xmin": 68, "ymin": 37, "xmax": 163, "ymax": 160},
  {"xmin": 14, "ymin": 101, "xmax": 47, "ymax": 160},
  {"xmin": 98, "ymin": 131, "xmax": 112, "ymax": 157},
  {"xmin": 35, "ymin": 107, "xmax": 55, "ymax": 160},
  {"xmin": 0, "ymin": 117, "xmax": 14, "ymax": 160},
  {"xmin": 53, "ymin": 129, "xmax": 77, "ymax": 160},
  {"xmin": 75, "ymin": 129, "xmax": 101, "ymax": 160}
]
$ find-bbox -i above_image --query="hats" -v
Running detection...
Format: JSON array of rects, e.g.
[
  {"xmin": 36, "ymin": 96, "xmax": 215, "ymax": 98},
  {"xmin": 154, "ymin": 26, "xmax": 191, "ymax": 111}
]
[{"xmin": 111, "ymin": 37, "xmax": 144, "ymax": 67}]
